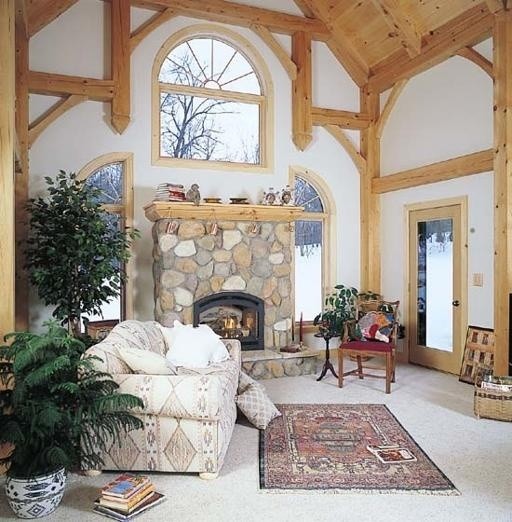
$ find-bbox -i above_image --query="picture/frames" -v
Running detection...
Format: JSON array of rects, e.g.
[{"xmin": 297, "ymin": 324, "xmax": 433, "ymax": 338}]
[{"xmin": 458, "ymin": 324, "xmax": 494, "ymax": 387}]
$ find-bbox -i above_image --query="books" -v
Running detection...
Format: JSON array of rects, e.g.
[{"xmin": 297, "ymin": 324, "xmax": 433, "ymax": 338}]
[
  {"xmin": 90, "ymin": 472, "xmax": 165, "ymax": 522},
  {"xmin": 155, "ymin": 183, "xmax": 186, "ymax": 202}
]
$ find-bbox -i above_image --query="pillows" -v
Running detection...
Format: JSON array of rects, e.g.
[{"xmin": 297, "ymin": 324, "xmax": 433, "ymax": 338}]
[
  {"xmin": 174, "ymin": 319, "xmax": 232, "ymax": 363},
  {"xmin": 238, "ymin": 369, "xmax": 267, "ymax": 398},
  {"xmin": 112, "ymin": 343, "xmax": 179, "ymax": 378},
  {"xmin": 234, "ymin": 383, "xmax": 283, "ymax": 430},
  {"xmin": 356, "ymin": 310, "xmax": 395, "ymax": 343},
  {"xmin": 159, "ymin": 325, "xmax": 222, "ymax": 371}
]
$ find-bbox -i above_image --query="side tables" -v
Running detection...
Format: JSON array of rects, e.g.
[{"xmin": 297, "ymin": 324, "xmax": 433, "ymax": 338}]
[{"xmin": 315, "ymin": 331, "xmax": 340, "ymax": 381}]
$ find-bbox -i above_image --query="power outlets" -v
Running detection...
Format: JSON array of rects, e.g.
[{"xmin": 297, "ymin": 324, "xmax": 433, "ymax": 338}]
[{"xmin": 472, "ymin": 273, "xmax": 484, "ymax": 288}]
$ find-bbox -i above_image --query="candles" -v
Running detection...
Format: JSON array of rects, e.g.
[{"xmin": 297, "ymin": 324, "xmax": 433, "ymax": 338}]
[
  {"xmin": 300, "ymin": 311, "xmax": 305, "ymax": 343},
  {"xmin": 278, "ymin": 332, "xmax": 282, "ymax": 347},
  {"xmin": 272, "ymin": 324, "xmax": 275, "ymax": 347},
  {"xmin": 291, "ymin": 316, "xmax": 295, "ymax": 344},
  {"xmin": 285, "ymin": 320, "xmax": 289, "ymax": 348}
]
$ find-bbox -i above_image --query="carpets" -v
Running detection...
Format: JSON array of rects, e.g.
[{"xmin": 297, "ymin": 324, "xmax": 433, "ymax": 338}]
[{"xmin": 257, "ymin": 401, "xmax": 464, "ymax": 499}]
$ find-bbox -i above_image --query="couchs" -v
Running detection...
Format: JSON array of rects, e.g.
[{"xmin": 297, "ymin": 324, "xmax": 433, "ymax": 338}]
[{"xmin": 78, "ymin": 318, "xmax": 243, "ymax": 481}]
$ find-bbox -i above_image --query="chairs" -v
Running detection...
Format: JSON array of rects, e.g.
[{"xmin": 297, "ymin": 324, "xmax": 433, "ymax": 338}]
[{"xmin": 337, "ymin": 297, "xmax": 402, "ymax": 395}]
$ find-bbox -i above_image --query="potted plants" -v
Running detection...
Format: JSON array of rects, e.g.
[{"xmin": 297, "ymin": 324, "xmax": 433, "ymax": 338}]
[
  {"xmin": 313, "ymin": 284, "xmax": 394, "ymax": 362},
  {"xmin": 0, "ymin": 312, "xmax": 146, "ymax": 519}
]
